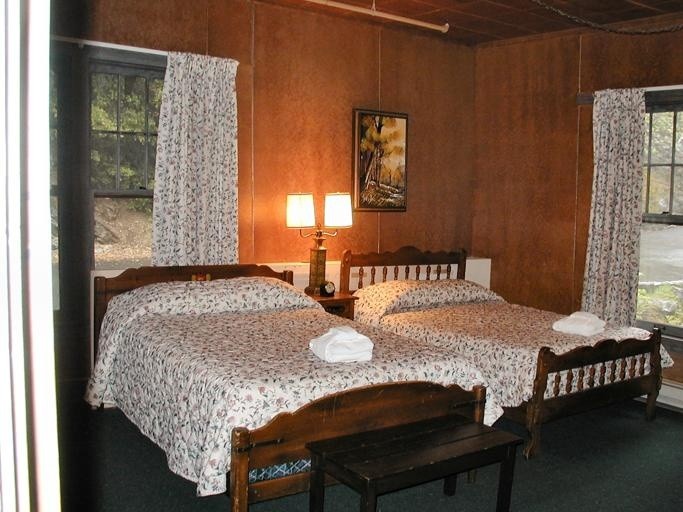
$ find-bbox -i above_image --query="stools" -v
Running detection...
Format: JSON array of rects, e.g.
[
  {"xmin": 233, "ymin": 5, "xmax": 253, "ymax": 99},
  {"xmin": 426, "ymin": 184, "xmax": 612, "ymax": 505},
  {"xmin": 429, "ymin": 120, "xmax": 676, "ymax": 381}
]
[{"xmin": 305, "ymin": 412, "xmax": 523, "ymax": 510}]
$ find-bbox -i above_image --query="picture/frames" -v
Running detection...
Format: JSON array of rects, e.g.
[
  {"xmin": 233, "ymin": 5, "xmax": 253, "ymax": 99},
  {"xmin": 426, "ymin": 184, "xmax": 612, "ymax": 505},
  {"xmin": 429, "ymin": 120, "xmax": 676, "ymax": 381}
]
[{"xmin": 352, "ymin": 110, "xmax": 408, "ymax": 212}]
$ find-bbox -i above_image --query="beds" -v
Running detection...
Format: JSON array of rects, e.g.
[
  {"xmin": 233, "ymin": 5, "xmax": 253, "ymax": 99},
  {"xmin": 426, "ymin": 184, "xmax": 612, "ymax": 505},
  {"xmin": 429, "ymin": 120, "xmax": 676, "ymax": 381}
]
[
  {"xmin": 93, "ymin": 265, "xmax": 503, "ymax": 510},
  {"xmin": 340, "ymin": 247, "xmax": 663, "ymax": 462}
]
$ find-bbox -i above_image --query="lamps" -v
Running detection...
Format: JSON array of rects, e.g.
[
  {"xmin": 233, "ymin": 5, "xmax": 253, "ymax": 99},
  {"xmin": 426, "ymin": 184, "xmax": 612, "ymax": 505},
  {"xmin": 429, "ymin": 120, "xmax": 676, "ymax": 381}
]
[{"xmin": 284, "ymin": 192, "xmax": 353, "ymax": 294}]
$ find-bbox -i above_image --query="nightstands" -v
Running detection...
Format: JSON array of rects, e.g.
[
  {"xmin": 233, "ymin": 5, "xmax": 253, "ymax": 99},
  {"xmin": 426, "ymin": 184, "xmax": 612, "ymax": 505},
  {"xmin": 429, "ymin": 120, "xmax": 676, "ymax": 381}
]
[{"xmin": 299, "ymin": 292, "xmax": 360, "ymax": 321}]
